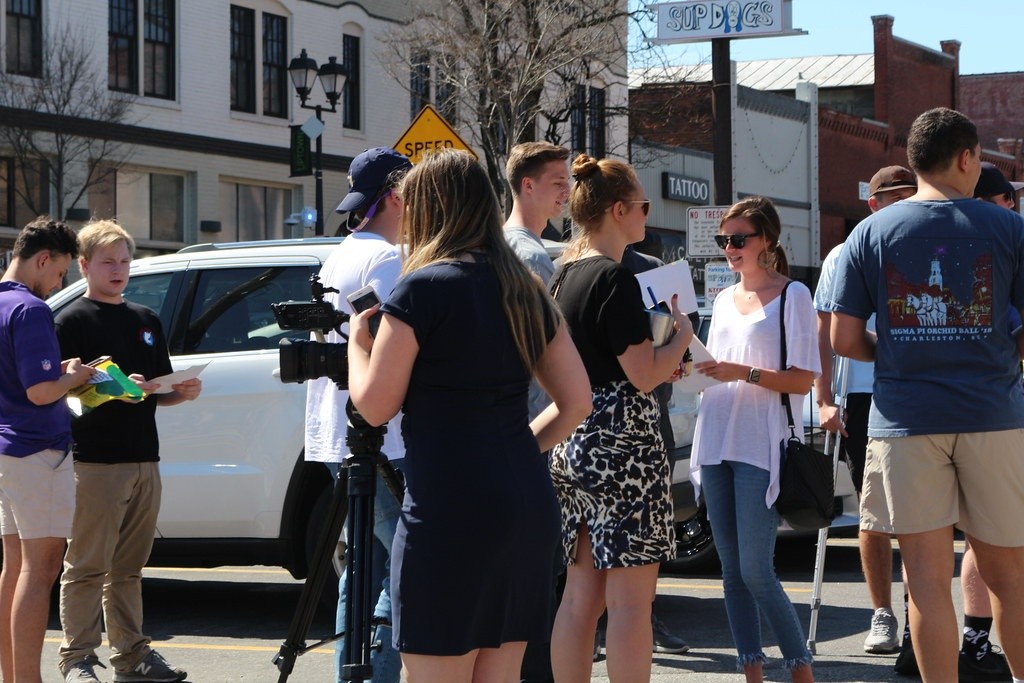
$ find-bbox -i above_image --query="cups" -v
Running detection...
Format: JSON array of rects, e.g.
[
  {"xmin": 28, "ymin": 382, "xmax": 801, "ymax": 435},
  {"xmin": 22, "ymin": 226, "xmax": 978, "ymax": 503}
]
[{"xmin": 644, "ymin": 309, "xmax": 674, "ymax": 349}]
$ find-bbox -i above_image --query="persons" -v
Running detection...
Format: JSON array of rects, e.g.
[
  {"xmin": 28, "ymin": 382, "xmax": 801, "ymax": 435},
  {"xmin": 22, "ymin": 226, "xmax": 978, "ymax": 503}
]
[
  {"xmin": 304, "ymin": 138, "xmax": 701, "ymax": 683},
  {"xmin": 812, "ymin": 106, "xmax": 1024, "ymax": 683},
  {"xmin": 689, "ymin": 195, "xmax": 822, "ymax": 683},
  {"xmin": 0, "ymin": 210, "xmax": 202, "ymax": 682}
]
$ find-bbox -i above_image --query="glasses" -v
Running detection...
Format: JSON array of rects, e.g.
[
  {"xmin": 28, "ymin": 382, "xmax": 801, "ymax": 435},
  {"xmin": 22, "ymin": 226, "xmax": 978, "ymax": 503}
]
[
  {"xmin": 1004, "ymin": 194, "xmax": 1011, "ymax": 202},
  {"xmin": 714, "ymin": 233, "xmax": 758, "ymax": 248},
  {"xmin": 605, "ymin": 198, "xmax": 652, "ymax": 215}
]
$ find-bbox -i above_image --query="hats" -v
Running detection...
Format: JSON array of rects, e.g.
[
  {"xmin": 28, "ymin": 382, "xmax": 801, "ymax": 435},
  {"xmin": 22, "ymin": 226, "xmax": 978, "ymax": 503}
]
[
  {"xmin": 337, "ymin": 147, "xmax": 411, "ymax": 213},
  {"xmin": 868, "ymin": 166, "xmax": 921, "ymax": 198},
  {"xmin": 974, "ymin": 161, "xmax": 1009, "ymax": 196}
]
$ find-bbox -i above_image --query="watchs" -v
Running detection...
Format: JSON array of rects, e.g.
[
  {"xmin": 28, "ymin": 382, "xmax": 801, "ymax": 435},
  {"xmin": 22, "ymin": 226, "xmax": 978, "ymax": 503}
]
[{"xmin": 747, "ymin": 368, "xmax": 760, "ymax": 383}]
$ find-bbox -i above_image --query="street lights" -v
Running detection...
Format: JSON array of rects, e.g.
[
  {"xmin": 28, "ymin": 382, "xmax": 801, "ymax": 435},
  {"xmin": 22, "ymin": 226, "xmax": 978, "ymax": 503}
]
[{"xmin": 287, "ymin": 49, "xmax": 348, "ymax": 235}]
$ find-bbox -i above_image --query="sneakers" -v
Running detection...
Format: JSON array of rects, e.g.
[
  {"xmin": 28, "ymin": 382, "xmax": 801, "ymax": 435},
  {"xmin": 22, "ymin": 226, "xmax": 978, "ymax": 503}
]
[
  {"xmin": 864, "ymin": 610, "xmax": 899, "ymax": 651},
  {"xmin": 651, "ymin": 618, "xmax": 689, "ymax": 653},
  {"xmin": 894, "ymin": 632, "xmax": 920, "ymax": 674},
  {"xmin": 958, "ymin": 647, "xmax": 1013, "ymax": 683},
  {"xmin": 64, "ymin": 659, "xmax": 107, "ymax": 683},
  {"xmin": 593, "ymin": 631, "xmax": 603, "ymax": 660},
  {"xmin": 115, "ymin": 650, "xmax": 187, "ymax": 683}
]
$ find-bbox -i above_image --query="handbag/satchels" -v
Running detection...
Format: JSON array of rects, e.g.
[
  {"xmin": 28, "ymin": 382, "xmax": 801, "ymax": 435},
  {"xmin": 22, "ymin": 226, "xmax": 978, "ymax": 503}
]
[{"xmin": 775, "ymin": 439, "xmax": 837, "ymax": 529}]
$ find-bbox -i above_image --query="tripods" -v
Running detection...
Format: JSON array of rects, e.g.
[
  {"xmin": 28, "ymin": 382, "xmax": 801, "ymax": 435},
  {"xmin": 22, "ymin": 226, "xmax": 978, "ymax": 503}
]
[{"xmin": 272, "ymin": 396, "xmax": 405, "ymax": 683}]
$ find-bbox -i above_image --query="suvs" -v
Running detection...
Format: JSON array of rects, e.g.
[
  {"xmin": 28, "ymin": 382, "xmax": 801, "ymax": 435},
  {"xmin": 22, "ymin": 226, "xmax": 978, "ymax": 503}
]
[{"xmin": 0, "ymin": 238, "xmax": 714, "ymax": 627}]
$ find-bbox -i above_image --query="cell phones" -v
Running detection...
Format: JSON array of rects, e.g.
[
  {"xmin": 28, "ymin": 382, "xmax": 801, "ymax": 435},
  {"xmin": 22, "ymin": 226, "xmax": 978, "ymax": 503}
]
[
  {"xmin": 347, "ymin": 285, "xmax": 384, "ymax": 340},
  {"xmin": 85, "ymin": 356, "xmax": 111, "ymax": 367}
]
